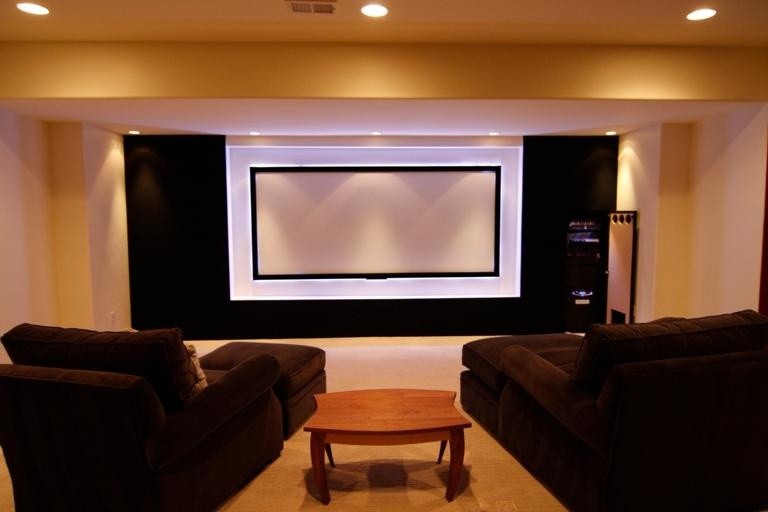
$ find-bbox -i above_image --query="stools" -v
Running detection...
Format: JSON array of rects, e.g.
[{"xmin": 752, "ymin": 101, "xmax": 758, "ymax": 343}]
[
  {"xmin": 199, "ymin": 342, "xmax": 326, "ymax": 441},
  {"xmin": 461, "ymin": 334, "xmax": 584, "ymax": 442}
]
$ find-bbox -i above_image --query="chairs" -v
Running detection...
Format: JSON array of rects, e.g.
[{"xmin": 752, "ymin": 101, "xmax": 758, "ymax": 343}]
[
  {"xmin": 0, "ymin": 354, "xmax": 283, "ymax": 512},
  {"xmin": 498, "ymin": 318, "xmax": 768, "ymax": 512}
]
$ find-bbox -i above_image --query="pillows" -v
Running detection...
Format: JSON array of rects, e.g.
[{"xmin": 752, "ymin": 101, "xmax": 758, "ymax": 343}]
[
  {"xmin": 120, "ymin": 328, "xmax": 208, "ymax": 393},
  {"xmin": 574, "ymin": 310, "xmax": 768, "ymax": 399},
  {"xmin": 0, "ymin": 323, "xmax": 198, "ymax": 413}
]
